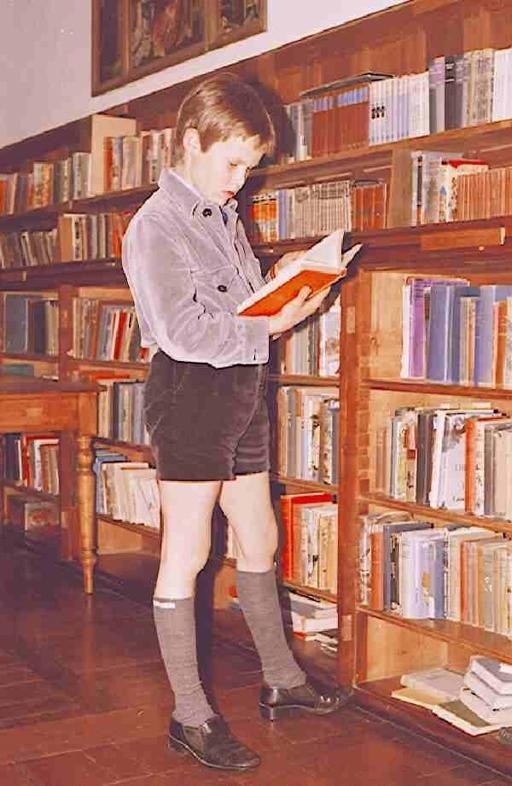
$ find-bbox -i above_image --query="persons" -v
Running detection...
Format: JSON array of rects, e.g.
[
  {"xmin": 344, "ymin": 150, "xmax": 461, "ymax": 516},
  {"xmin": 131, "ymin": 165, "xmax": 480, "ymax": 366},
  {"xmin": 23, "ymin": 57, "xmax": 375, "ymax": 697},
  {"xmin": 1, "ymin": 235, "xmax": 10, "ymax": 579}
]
[{"xmin": 121, "ymin": 71, "xmax": 350, "ymax": 771}]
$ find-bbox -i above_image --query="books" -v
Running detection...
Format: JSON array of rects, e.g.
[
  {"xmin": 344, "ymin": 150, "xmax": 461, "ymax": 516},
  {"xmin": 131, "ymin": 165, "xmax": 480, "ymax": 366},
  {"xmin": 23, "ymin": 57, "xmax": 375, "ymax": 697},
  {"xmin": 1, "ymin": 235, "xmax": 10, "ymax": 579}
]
[
  {"xmin": 208, "ymin": 47, "xmax": 512, "ymax": 739},
  {"xmin": 0, "ymin": 112, "xmax": 176, "ymax": 534}
]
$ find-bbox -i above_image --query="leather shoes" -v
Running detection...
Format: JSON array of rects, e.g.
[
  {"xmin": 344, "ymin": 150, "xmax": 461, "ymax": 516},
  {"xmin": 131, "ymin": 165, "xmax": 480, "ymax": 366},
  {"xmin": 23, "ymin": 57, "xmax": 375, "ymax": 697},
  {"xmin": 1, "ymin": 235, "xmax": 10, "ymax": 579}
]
[
  {"xmin": 260, "ymin": 676, "xmax": 354, "ymax": 720},
  {"xmin": 168, "ymin": 715, "xmax": 262, "ymax": 771}
]
